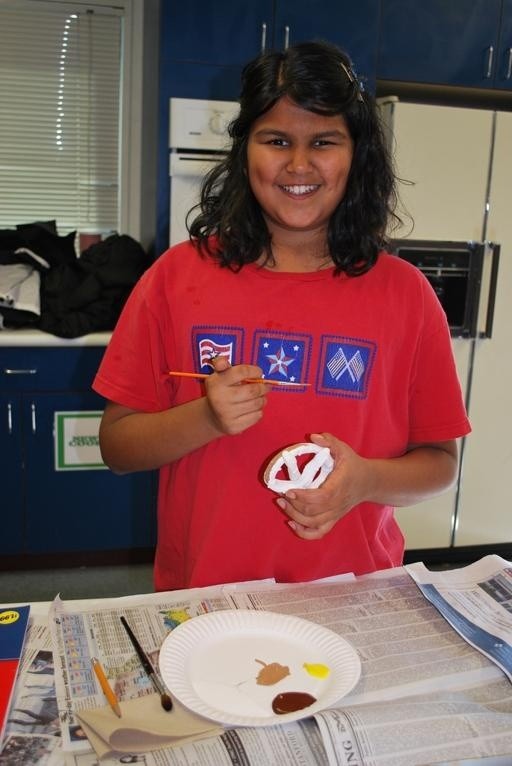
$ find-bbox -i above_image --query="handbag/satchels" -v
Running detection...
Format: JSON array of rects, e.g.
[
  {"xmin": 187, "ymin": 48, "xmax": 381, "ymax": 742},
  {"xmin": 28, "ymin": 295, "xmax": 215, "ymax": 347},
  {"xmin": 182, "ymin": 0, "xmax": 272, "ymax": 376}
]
[{"xmin": 0, "ymin": 226, "xmax": 150, "ymax": 338}]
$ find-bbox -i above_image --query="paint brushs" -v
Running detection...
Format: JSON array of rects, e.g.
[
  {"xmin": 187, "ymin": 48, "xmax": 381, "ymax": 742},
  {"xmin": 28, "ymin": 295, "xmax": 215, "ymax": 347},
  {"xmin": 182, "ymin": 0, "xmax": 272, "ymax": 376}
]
[
  {"xmin": 120, "ymin": 616, "xmax": 172, "ymax": 711},
  {"xmin": 168, "ymin": 371, "xmax": 311, "ymax": 387}
]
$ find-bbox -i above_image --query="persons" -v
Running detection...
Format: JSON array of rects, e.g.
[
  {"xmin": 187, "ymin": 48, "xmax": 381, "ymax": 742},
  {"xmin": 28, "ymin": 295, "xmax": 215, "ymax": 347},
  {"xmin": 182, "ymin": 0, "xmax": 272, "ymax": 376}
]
[{"xmin": 93, "ymin": 32, "xmax": 469, "ymax": 601}]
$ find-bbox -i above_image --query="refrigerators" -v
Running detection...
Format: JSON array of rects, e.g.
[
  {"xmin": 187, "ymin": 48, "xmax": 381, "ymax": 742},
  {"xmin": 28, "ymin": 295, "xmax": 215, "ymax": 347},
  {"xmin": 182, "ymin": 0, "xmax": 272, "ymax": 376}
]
[{"xmin": 371, "ymin": 92, "xmax": 512, "ymax": 552}]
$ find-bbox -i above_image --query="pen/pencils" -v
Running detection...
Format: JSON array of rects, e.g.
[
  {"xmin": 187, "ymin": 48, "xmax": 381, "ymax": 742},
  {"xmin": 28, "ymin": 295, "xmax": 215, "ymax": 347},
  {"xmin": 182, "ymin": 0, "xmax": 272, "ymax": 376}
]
[{"xmin": 90, "ymin": 657, "xmax": 122, "ymax": 719}]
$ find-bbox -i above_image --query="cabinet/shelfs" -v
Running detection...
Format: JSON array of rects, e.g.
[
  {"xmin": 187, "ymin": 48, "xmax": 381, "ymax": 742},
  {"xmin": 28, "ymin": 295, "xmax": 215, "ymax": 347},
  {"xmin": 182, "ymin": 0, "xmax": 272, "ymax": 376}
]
[
  {"xmin": 2, "ymin": 346, "xmax": 159, "ymax": 555},
  {"xmin": 376, "ymin": 80, "xmax": 511, "ymax": 563}
]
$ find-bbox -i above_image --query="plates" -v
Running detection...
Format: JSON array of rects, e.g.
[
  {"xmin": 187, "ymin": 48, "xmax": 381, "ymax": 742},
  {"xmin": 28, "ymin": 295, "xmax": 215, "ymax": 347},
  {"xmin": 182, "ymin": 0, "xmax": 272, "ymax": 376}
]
[{"xmin": 157, "ymin": 608, "xmax": 362, "ymax": 730}]
